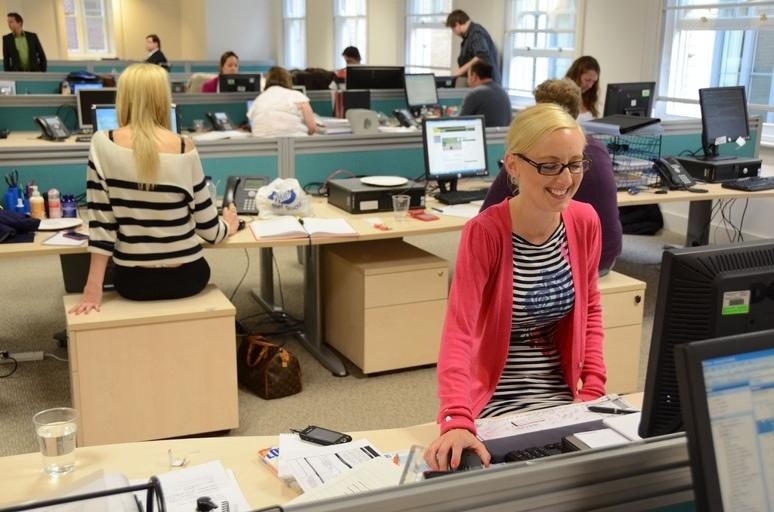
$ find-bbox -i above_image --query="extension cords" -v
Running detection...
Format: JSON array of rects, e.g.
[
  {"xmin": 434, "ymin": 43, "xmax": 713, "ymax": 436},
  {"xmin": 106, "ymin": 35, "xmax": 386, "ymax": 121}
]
[{"xmin": 0, "ymin": 350, "xmax": 44, "ymax": 364}]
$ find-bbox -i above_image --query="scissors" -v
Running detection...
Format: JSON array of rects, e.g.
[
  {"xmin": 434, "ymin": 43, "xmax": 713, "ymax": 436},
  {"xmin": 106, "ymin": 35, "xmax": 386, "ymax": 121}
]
[{"xmin": 4, "ymin": 168, "xmax": 19, "ymax": 188}]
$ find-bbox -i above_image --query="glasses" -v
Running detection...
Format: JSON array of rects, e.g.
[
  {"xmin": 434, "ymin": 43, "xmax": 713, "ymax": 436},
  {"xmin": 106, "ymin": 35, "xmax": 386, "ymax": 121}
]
[{"xmin": 517, "ymin": 153, "xmax": 593, "ymax": 177}]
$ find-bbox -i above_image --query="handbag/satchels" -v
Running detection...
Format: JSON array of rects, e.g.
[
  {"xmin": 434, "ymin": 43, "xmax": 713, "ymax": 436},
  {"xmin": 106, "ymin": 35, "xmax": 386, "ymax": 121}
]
[{"xmin": 237, "ymin": 333, "xmax": 302, "ymax": 400}]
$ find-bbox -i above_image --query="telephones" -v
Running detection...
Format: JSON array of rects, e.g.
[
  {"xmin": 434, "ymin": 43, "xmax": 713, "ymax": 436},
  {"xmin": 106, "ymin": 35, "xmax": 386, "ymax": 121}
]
[
  {"xmin": 392, "ymin": 108, "xmax": 419, "ymax": 128},
  {"xmin": 650, "ymin": 155, "xmax": 709, "ymax": 192},
  {"xmin": 222, "ymin": 176, "xmax": 268, "ymax": 215},
  {"xmin": 32, "ymin": 114, "xmax": 70, "ymax": 140},
  {"xmin": 204, "ymin": 111, "xmax": 236, "ymax": 131}
]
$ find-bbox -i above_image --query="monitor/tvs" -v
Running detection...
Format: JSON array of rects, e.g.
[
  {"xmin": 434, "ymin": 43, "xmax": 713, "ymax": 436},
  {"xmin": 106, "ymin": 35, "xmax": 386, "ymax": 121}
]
[
  {"xmin": 404, "ymin": 73, "xmax": 439, "ymax": 107},
  {"xmin": 422, "ymin": 115, "xmax": 489, "ymax": 195},
  {"xmin": 91, "ymin": 104, "xmax": 181, "ymax": 134},
  {"xmin": 74, "ymin": 82, "xmax": 104, "ymax": 94},
  {"xmin": 291, "ymin": 85, "xmax": 306, "ymax": 97},
  {"xmin": 674, "ymin": 329, "xmax": 774, "ymax": 512},
  {"xmin": 639, "ymin": 238, "xmax": 774, "ymax": 438},
  {"xmin": 347, "ymin": 66, "xmax": 405, "ymax": 89},
  {"xmin": 77, "ymin": 88, "xmax": 118, "ymax": 129},
  {"xmin": 696, "ymin": 86, "xmax": 751, "ymax": 161},
  {"xmin": 436, "ymin": 77, "xmax": 456, "ymax": 88},
  {"xmin": 220, "ymin": 74, "xmax": 260, "ymax": 92},
  {"xmin": 604, "ymin": 82, "xmax": 656, "ymax": 117}
]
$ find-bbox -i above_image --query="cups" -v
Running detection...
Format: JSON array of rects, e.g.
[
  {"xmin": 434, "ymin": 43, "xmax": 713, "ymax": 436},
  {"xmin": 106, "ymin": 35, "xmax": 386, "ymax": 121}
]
[
  {"xmin": 193, "ymin": 120, "xmax": 203, "ymax": 134},
  {"xmin": 392, "ymin": 195, "xmax": 409, "ymax": 220},
  {"xmin": 31, "ymin": 405, "xmax": 78, "ymax": 473}
]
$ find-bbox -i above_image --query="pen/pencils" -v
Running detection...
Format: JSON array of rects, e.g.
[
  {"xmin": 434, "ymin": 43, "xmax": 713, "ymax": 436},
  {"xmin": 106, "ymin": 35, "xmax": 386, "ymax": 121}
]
[
  {"xmin": 19, "ymin": 180, "xmax": 35, "ymax": 200},
  {"xmin": 298, "ymin": 218, "xmax": 307, "ymax": 232},
  {"xmin": 134, "ymin": 494, "xmax": 142, "ymax": 512},
  {"xmin": 432, "ymin": 208, "xmax": 443, "ymax": 212},
  {"xmin": 61, "ymin": 192, "xmax": 75, "ymax": 202},
  {"xmin": 587, "ymin": 406, "xmax": 641, "ymax": 414}
]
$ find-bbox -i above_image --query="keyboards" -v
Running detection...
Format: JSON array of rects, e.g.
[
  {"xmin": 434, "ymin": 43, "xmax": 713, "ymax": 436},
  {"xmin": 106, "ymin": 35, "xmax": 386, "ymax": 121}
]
[
  {"xmin": 435, "ymin": 190, "xmax": 488, "ymax": 205},
  {"xmin": 505, "ymin": 443, "xmax": 575, "ymax": 462},
  {"xmin": 76, "ymin": 136, "xmax": 91, "ymax": 142},
  {"xmin": 722, "ymin": 176, "xmax": 774, "ymax": 191}
]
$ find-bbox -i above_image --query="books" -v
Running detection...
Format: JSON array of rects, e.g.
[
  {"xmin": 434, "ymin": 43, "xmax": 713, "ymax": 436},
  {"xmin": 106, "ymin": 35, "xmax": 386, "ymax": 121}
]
[
  {"xmin": 2, "ymin": 462, "xmax": 255, "ymax": 512},
  {"xmin": 259, "ymin": 431, "xmax": 423, "ymax": 512},
  {"xmin": 608, "ymin": 153, "xmax": 660, "ymax": 191},
  {"xmin": 247, "ymin": 215, "xmax": 360, "ymax": 241},
  {"xmin": 316, "ymin": 114, "xmax": 353, "ymax": 136}
]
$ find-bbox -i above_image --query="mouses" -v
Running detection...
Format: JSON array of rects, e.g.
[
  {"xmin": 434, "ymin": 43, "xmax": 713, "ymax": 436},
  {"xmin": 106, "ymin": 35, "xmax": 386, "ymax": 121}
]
[
  {"xmin": 238, "ymin": 219, "xmax": 245, "ymax": 230},
  {"xmin": 443, "ymin": 447, "xmax": 482, "ymax": 472}
]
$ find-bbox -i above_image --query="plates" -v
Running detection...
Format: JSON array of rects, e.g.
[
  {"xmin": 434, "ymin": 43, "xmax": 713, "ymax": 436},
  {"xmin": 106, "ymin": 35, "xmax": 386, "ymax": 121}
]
[{"xmin": 35, "ymin": 217, "xmax": 83, "ymax": 231}]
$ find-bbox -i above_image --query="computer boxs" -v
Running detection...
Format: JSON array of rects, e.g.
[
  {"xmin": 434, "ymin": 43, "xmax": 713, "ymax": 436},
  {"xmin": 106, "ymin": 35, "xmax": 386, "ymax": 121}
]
[
  {"xmin": 328, "ymin": 175, "xmax": 426, "ymax": 214},
  {"xmin": 674, "ymin": 154, "xmax": 762, "ymax": 182}
]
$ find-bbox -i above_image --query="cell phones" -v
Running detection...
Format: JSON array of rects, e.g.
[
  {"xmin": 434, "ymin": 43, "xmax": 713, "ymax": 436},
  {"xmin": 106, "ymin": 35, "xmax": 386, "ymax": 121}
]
[
  {"xmin": 64, "ymin": 232, "xmax": 89, "ymax": 240},
  {"xmin": 290, "ymin": 425, "xmax": 352, "ymax": 445}
]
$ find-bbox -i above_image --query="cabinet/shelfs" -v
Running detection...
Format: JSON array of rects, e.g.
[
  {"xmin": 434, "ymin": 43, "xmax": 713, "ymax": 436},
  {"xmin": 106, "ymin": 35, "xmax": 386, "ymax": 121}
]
[{"xmin": 598, "ymin": 268, "xmax": 649, "ymax": 397}]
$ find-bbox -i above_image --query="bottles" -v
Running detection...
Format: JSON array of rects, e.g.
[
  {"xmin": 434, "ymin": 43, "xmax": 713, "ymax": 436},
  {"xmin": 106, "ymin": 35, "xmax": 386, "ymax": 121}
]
[{"xmin": 5, "ymin": 184, "xmax": 75, "ymax": 219}]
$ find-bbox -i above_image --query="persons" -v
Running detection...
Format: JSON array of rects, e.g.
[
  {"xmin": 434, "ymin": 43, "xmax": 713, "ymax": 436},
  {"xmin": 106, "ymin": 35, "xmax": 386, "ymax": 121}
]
[
  {"xmin": 453, "ymin": 60, "xmax": 512, "ymax": 129},
  {"xmin": 64, "ymin": 63, "xmax": 240, "ymax": 315},
  {"xmin": 444, "ymin": 8, "xmax": 503, "ymax": 87},
  {"xmin": 134, "ymin": 33, "xmax": 168, "ymax": 70},
  {"xmin": 1, "ymin": 11, "xmax": 48, "ymax": 71},
  {"xmin": 565, "ymin": 55, "xmax": 603, "ymax": 122},
  {"xmin": 334, "ymin": 45, "xmax": 362, "ymax": 78},
  {"xmin": 244, "ymin": 65, "xmax": 317, "ymax": 140},
  {"xmin": 478, "ymin": 78, "xmax": 626, "ymax": 285},
  {"xmin": 416, "ymin": 98, "xmax": 610, "ymax": 473},
  {"xmin": 199, "ymin": 52, "xmax": 240, "ymax": 93}
]
